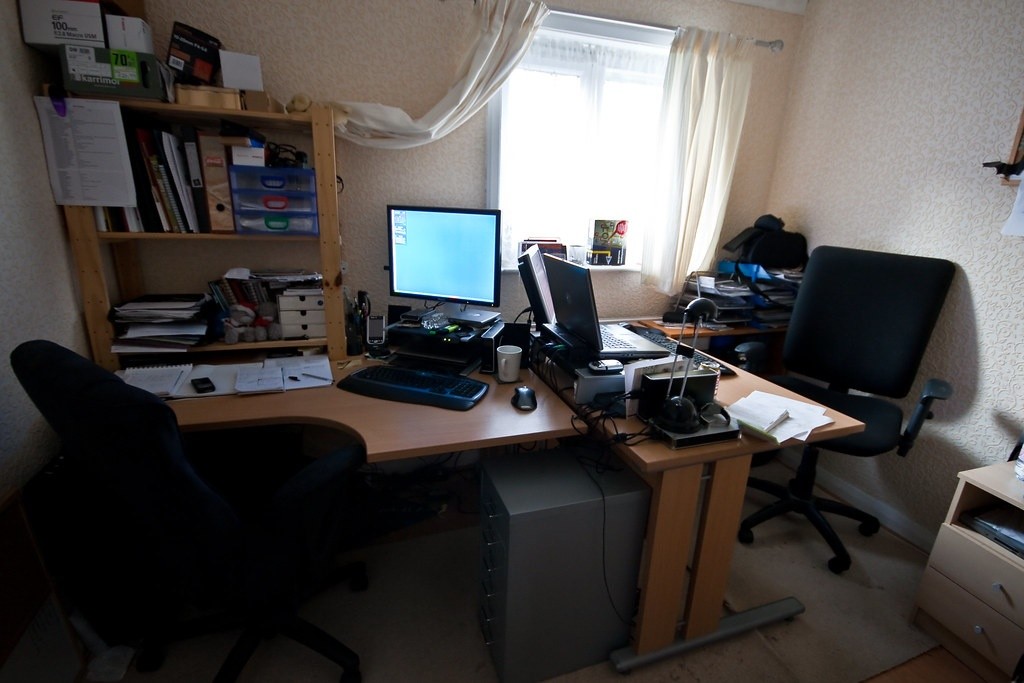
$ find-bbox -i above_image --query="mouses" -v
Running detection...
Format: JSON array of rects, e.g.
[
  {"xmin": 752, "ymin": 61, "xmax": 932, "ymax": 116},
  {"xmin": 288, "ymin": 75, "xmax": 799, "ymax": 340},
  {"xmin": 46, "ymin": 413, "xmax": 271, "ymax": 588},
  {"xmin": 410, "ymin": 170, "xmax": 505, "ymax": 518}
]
[{"xmin": 510, "ymin": 386, "xmax": 537, "ymax": 410}]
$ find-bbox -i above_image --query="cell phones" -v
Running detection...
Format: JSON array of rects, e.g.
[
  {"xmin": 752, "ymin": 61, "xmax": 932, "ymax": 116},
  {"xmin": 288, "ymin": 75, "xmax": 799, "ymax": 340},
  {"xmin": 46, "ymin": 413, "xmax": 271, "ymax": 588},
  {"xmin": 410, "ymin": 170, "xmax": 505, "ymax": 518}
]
[
  {"xmin": 191, "ymin": 378, "xmax": 215, "ymax": 394},
  {"xmin": 367, "ymin": 316, "xmax": 385, "ymax": 344}
]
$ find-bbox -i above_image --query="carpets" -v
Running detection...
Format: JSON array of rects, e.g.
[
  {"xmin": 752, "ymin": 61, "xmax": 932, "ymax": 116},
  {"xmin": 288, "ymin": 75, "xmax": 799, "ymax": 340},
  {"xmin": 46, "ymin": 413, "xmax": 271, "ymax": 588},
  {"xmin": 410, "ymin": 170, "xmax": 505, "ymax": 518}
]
[{"xmin": 80, "ymin": 463, "xmax": 943, "ymax": 682}]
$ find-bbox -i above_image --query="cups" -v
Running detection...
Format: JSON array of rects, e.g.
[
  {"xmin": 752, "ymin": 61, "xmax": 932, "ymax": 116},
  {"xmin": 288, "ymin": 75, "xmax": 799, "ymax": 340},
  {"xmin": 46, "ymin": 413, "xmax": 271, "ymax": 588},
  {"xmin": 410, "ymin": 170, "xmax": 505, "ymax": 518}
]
[{"xmin": 497, "ymin": 345, "xmax": 522, "ymax": 382}]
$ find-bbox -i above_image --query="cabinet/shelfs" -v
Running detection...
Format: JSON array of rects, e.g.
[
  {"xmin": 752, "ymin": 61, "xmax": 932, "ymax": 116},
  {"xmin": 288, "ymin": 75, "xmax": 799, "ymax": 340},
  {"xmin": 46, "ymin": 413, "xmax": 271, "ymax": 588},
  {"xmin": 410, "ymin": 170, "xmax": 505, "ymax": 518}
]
[
  {"xmin": 482, "ymin": 455, "xmax": 713, "ymax": 682},
  {"xmin": 37, "ymin": 77, "xmax": 349, "ymax": 362},
  {"xmin": 906, "ymin": 460, "xmax": 1024, "ymax": 683}
]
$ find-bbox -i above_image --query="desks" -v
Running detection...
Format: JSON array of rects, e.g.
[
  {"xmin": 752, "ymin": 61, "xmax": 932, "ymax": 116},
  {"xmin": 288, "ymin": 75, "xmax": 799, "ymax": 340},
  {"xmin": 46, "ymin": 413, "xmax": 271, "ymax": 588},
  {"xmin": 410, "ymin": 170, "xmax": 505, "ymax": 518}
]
[
  {"xmin": 543, "ymin": 323, "xmax": 865, "ymax": 672},
  {"xmin": 112, "ymin": 353, "xmax": 587, "ymax": 461}
]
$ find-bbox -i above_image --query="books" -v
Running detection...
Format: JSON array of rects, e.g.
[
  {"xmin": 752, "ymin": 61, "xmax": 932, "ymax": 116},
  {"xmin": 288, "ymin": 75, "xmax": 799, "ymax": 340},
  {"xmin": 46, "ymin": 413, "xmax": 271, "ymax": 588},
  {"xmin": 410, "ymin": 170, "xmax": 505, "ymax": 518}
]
[
  {"xmin": 107, "ymin": 291, "xmax": 215, "ymax": 354},
  {"xmin": 112, "ymin": 362, "xmax": 265, "ymax": 400},
  {"xmin": 264, "ymin": 268, "xmax": 325, "ymax": 296},
  {"xmin": 234, "ymin": 358, "xmax": 333, "ymax": 394},
  {"xmin": 207, "ymin": 273, "xmax": 271, "ymax": 311},
  {"xmin": 90, "ymin": 124, "xmax": 214, "ymax": 234},
  {"xmin": 521, "ymin": 238, "xmax": 568, "ymax": 261},
  {"xmin": 726, "ymin": 396, "xmax": 790, "ymax": 432}
]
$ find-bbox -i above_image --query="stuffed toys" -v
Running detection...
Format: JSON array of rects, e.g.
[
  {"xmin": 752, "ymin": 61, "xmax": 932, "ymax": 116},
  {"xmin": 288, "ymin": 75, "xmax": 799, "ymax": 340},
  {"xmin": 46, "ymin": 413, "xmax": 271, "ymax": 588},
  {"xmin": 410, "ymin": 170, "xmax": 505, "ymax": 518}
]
[
  {"xmin": 221, "ymin": 301, "xmax": 257, "ymax": 344},
  {"xmin": 252, "ymin": 304, "xmax": 282, "ymax": 341}
]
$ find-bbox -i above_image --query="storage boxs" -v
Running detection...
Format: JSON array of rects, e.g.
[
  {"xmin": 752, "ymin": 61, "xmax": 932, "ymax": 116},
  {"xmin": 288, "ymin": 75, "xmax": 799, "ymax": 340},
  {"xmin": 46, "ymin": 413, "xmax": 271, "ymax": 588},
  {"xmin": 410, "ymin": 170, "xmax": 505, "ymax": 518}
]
[
  {"xmin": 278, "ymin": 293, "xmax": 327, "ymax": 341},
  {"xmin": 17, "ymin": 0, "xmax": 244, "ymax": 112},
  {"xmin": 228, "ymin": 165, "xmax": 319, "ymax": 236}
]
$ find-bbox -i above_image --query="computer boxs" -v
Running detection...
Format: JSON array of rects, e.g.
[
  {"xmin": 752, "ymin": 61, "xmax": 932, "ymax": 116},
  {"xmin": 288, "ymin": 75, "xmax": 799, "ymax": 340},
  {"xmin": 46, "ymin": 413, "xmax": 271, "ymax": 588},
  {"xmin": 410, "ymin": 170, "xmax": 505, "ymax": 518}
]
[{"xmin": 384, "ymin": 321, "xmax": 486, "ymax": 365}]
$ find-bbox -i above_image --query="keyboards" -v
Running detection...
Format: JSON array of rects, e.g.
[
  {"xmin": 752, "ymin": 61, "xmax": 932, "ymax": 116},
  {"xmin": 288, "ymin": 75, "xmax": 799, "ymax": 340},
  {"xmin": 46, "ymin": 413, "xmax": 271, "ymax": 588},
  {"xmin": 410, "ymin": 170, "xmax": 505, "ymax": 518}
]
[
  {"xmin": 640, "ymin": 333, "xmax": 738, "ymax": 376},
  {"xmin": 337, "ymin": 365, "xmax": 489, "ymax": 411}
]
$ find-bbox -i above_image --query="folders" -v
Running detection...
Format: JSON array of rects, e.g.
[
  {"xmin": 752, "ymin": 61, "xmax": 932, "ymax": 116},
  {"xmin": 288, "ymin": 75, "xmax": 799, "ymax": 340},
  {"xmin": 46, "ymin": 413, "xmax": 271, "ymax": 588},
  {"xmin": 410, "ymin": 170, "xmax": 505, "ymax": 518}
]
[{"xmin": 124, "ymin": 129, "xmax": 164, "ymax": 233}]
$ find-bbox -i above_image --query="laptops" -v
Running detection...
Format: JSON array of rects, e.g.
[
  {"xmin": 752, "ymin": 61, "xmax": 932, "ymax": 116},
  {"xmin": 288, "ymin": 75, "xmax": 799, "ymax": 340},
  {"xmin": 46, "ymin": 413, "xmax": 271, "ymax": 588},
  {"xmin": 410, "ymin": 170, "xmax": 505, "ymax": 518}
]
[{"xmin": 543, "ymin": 252, "xmax": 670, "ymax": 359}]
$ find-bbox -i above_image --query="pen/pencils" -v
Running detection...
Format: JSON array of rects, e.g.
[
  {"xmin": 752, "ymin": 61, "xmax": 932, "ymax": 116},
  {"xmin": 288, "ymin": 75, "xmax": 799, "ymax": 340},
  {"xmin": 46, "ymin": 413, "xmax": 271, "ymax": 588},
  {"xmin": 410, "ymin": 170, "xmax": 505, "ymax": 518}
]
[{"xmin": 301, "ymin": 373, "xmax": 336, "ymax": 382}]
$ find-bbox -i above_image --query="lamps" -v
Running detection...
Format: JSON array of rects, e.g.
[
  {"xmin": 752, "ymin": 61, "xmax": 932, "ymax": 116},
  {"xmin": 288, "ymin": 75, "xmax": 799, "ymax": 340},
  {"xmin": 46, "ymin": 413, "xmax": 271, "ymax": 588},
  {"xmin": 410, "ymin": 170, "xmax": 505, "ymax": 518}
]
[{"xmin": 656, "ymin": 298, "xmax": 717, "ymax": 433}]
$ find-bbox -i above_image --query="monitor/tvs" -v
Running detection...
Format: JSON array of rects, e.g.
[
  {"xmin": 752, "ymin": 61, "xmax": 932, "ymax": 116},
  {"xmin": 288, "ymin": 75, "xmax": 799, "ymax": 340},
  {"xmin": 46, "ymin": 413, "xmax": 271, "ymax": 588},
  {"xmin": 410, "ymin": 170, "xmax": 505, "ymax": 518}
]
[
  {"xmin": 517, "ymin": 243, "xmax": 556, "ymax": 331},
  {"xmin": 386, "ymin": 205, "xmax": 501, "ymax": 310}
]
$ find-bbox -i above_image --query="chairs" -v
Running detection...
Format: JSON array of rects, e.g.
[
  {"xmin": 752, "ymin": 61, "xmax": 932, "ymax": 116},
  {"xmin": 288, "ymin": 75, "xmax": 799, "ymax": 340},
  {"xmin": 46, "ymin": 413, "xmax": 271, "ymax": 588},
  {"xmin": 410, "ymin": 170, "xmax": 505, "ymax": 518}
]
[
  {"xmin": 10, "ymin": 332, "xmax": 367, "ymax": 683},
  {"xmin": 728, "ymin": 245, "xmax": 956, "ymax": 575}
]
